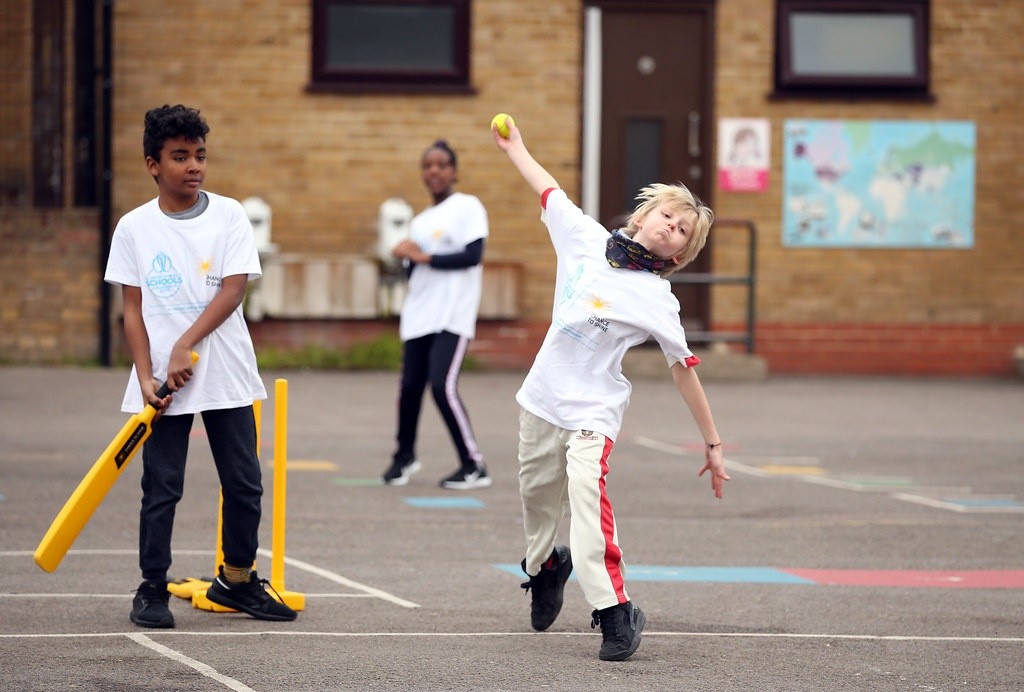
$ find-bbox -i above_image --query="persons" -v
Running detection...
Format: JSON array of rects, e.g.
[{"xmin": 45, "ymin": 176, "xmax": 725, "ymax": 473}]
[
  {"xmin": 493, "ymin": 115, "xmax": 732, "ymax": 660},
  {"xmin": 379, "ymin": 140, "xmax": 492, "ymax": 490},
  {"xmin": 103, "ymin": 105, "xmax": 299, "ymax": 628}
]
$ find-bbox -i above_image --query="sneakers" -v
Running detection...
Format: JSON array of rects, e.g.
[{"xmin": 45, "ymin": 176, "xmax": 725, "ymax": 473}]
[
  {"xmin": 442, "ymin": 463, "xmax": 492, "ymax": 488},
  {"xmin": 520, "ymin": 545, "xmax": 574, "ymax": 630},
  {"xmin": 129, "ymin": 581, "xmax": 175, "ymax": 628},
  {"xmin": 385, "ymin": 459, "xmax": 421, "ymax": 484},
  {"xmin": 205, "ymin": 565, "xmax": 297, "ymax": 621},
  {"xmin": 592, "ymin": 600, "xmax": 642, "ymax": 660}
]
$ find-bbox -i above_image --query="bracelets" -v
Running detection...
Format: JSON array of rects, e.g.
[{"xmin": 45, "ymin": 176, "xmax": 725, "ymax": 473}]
[{"xmin": 706, "ymin": 442, "xmax": 722, "ymax": 448}]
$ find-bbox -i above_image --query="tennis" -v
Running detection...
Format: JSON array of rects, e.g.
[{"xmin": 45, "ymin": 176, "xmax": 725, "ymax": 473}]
[{"xmin": 490, "ymin": 112, "xmax": 515, "ymax": 138}]
